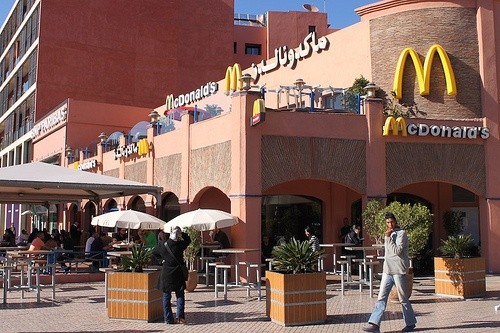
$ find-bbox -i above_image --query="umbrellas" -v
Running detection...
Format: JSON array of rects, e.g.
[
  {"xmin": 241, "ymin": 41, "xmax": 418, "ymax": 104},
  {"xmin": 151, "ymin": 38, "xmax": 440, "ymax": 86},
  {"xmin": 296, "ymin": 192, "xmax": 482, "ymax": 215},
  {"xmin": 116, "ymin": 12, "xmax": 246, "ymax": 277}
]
[
  {"xmin": 91, "ymin": 208, "xmax": 167, "ymax": 251},
  {"xmin": 164, "ymin": 207, "xmax": 239, "ymax": 270},
  {"xmin": 264, "ymin": 195, "xmax": 312, "ymax": 205}
]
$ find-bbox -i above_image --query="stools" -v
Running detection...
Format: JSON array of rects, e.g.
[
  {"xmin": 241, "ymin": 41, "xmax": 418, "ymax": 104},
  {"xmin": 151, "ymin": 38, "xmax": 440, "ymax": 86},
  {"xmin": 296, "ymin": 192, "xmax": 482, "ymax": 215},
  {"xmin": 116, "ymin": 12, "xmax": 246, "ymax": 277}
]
[
  {"xmin": 0, "ymin": 258, "xmax": 60, "ymax": 308},
  {"xmin": 200, "ymin": 255, "xmax": 386, "ymax": 303}
]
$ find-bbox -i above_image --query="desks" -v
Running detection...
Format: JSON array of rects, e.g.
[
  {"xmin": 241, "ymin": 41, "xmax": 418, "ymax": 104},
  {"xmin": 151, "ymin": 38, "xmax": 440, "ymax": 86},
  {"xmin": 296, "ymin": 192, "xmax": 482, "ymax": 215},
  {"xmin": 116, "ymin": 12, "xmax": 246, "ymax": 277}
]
[
  {"xmin": 203, "ymin": 244, "xmax": 220, "ymax": 257},
  {"xmin": 0, "ymin": 247, "xmax": 28, "ymax": 250},
  {"xmin": 319, "ymin": 243, "xmax": 356, "ymax": 275},
  {"xmin": 99, "ymin": 267, "xmax": 157, "ymax": 306},
  {"xmin": 8, "ymin": 250, "xmax": 52, "ymax": 260},
  {"xmin": 107, "ymin": 250, "xmax": 142, "ymax": 256},
  {"xmin": 213, "ymin": 247, "xmax": 259, "ymax": 285},
  {"xmin": 343, "ymin": 246, "xmax": 383, "ymax": 282}
]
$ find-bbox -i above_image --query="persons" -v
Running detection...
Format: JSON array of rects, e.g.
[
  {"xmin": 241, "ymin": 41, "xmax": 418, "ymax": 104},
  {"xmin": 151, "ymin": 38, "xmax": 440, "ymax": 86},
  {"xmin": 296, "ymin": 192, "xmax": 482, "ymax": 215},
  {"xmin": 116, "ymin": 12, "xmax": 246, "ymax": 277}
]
[
  {"xmin": 3, "ymin": 221, "xmax": 164, "ymax": 324},
  {"xmin": 151, "ymin": 226, "xmax": 192, "ymax": 324},
  {"xmin": 198, "ymin": 223, "xmax": 230, "ymax": 273},
  {"xmin": 340, "ymin": 217, "xmax": 365, "ymax": 259},
  {"xmin": 304, "ymin": 226, "xmax": 320, "ymax": 252},
  {"xmin": 363, "ymin": 211, "xmax": 417, "ymax": 333}
]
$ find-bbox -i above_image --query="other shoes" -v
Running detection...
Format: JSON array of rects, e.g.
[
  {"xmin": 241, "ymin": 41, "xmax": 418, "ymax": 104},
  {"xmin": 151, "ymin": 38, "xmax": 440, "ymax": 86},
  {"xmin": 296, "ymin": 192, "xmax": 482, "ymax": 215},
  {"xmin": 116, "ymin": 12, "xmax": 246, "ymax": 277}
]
[
  {"xmin": 402, "ymin": 324, "xmax": 416, "ymax": 332},
  {"xmin": 164, "ymin": 316, "xmax": 174, "ymax": 324},
  {"xmin": 176, "ymin": 317, "xmax": 185, "ymax": 323},
  {"xmin": 362, "ymin": 324, "xmax": 380, "ymax": 333}
]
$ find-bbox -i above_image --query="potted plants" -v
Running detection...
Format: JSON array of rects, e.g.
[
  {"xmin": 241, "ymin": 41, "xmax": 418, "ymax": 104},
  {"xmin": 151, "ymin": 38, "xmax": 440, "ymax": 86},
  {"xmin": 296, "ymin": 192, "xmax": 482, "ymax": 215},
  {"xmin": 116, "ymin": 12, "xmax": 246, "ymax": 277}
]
[
  {"xmin": 180, "ymin": 227, "xmax": 204, "ymax": 291},
  {"xmin": 107, "ymin": 245, "xmax": 162, "ymax": 320},
  {"xmin": 264, "ymin": 237, "xmax": 329, "ymax": 324},
  {"xmin": 434, "ymin": 234, "xmax": 485, "ymax": 298},
  {"xmin": 376, "ymin": 202, "xmax": 435, "ymax": 304}
]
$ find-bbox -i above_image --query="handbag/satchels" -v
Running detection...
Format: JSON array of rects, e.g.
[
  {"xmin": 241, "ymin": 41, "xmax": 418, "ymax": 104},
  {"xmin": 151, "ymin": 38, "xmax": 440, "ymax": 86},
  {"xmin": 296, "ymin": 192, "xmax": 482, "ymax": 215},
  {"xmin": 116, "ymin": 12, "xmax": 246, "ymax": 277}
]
[{"xmin": 164, "ymin": 241, "xmax": 188, "ymax": 281}]
